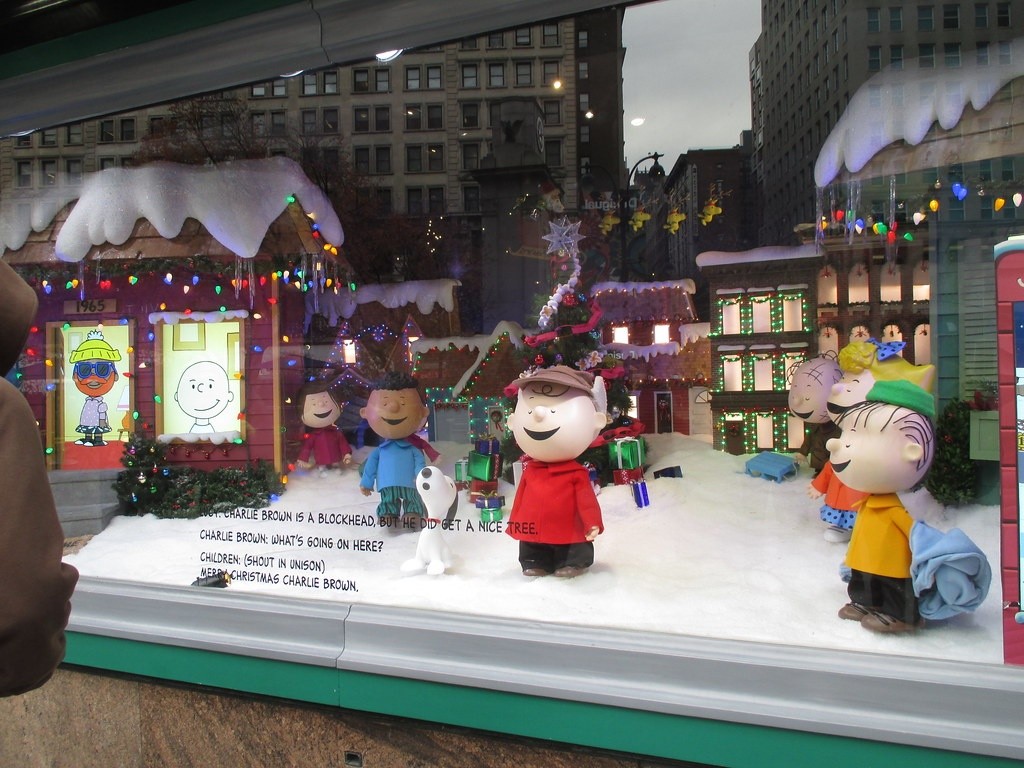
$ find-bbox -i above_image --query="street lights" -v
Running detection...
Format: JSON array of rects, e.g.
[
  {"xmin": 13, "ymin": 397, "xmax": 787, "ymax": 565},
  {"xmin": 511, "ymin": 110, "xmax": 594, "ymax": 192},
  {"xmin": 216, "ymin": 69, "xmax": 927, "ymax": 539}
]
[{"xmin": 618, "ymin": 153, "xmax": 666, "ymax": 281}]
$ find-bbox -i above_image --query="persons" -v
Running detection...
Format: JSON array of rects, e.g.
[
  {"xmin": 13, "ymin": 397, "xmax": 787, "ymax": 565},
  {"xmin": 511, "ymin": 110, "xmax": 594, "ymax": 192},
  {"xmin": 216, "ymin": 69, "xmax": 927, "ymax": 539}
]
[
  {"xmin": 358, "ymin": 375, "xmax": 442, "ymax": 535},
  {"xmin": 786, "ymin": 349, "xmax": 844, "ymax": 477},
  {"xmin": 297, "ymin": 381, "xmax": 352, "ymax": 469},
  {"xmin": 825, "ymin": 378, "xmax": 992, "ymax": 633},
  {"xmin": 0, "ymin": 261, "xmax": 79, "ymax": 701},
  {"xmin": 807, "ymin": 338, "xmax": 936, "ymax": 542},
  {"xmin": 506, "ymin": 365, "xmax": 607, "ymax": 577}
]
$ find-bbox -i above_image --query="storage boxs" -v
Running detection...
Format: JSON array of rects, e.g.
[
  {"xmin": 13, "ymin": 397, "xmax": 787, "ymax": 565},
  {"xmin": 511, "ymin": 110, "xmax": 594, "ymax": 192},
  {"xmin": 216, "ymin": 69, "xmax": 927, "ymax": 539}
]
[
  {"xmin": 652, "ymin": 466, "xmax": 684, "ymax": 480},
  {"xmin": 512, "ymin": 459, "xmax": 529, "ymax": 490},
  {"xmin": 607, "ymin": 435, "xmax": 650, "ymax": 508},
  {"xmin": 452, "ymin": 435, "xmax": 506, "ymax": 521}
]
